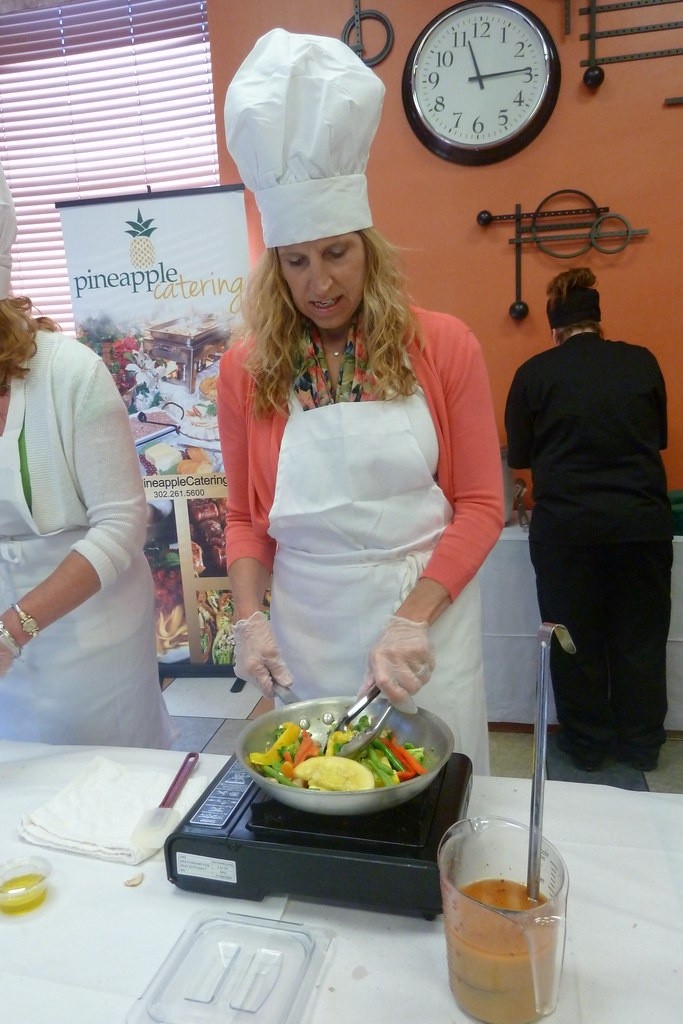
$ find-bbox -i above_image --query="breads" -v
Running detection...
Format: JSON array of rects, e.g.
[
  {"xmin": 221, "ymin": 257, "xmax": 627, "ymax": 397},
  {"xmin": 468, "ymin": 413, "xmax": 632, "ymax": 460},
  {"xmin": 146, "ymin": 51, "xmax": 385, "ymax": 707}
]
[{"xmin": 178, "ymin": 449, "xmax": 212, "ymax": 474}]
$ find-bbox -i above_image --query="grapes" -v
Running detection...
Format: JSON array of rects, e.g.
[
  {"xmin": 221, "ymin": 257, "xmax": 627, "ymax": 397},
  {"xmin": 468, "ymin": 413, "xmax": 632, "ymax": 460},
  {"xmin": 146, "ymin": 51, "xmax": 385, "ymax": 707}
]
[{"xmin": 139, "ymin": 454, "xmax": 158, "ymax": 476}]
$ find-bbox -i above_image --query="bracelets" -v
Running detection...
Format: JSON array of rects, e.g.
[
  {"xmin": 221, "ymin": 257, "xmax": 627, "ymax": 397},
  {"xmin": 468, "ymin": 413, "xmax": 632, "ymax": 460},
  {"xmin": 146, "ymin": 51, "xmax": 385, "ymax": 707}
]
[{"xmin": 0, "ymin": 621, "xmax": 21, "ymax": 657}]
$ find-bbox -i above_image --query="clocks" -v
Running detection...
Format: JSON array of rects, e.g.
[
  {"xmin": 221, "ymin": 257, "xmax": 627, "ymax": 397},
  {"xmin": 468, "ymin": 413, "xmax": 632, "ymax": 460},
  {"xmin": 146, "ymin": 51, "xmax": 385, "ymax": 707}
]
[{"xmin": 401, "ymin": 0, "xmax": 561, "ymax": 167}]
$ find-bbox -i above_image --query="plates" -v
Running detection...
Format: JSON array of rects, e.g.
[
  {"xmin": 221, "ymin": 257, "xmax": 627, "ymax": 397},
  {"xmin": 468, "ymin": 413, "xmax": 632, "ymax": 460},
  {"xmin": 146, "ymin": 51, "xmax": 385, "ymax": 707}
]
[
  {"xmin": 129, "ymin": 407, "xmax": 183, "ymax": 445},
  {"xmin": 125, "ymin": 909, "xmax": 336, "ymax": 1024},
  {"xmin": 0, "ymin": 860, "xmax": 53, "ymax": 915}
]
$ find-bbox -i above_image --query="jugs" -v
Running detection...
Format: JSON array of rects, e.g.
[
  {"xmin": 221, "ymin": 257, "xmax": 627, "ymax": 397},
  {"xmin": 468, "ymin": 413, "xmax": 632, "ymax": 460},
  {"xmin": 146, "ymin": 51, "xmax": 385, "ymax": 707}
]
[{"xmin": 438, "ymin": 817, "xmax": 568, "ymax": 1024}]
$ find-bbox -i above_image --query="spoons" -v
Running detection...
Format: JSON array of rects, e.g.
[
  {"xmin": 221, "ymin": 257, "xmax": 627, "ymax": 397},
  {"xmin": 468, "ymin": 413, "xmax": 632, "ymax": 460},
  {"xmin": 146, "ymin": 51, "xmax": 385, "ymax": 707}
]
[{"xmin": 138, "ymin": 412, "xmax": 177, "ymax": 428}]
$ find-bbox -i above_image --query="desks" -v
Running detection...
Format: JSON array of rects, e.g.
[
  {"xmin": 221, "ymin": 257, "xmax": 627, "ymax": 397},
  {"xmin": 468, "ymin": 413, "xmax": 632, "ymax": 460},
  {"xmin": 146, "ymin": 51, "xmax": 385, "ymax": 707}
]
[
  {"xmin": 0, "ymin": 736, "xmax": 683, "ymax": 1024},
  {"xmin": 481, "ymin": 509, "xmax": 683, "ymax": 738}
]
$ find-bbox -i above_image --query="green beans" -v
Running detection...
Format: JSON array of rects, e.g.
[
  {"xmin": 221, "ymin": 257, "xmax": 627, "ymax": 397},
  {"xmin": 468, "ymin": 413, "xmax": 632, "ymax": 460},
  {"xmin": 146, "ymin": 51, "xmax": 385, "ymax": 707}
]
[
  {"xmin": 368, "ymin": 739, "xmax": 405, "ymax": 788},
  {"xmin": 259, "ymin": 765, "xmax": 298, "ymax": 787}
]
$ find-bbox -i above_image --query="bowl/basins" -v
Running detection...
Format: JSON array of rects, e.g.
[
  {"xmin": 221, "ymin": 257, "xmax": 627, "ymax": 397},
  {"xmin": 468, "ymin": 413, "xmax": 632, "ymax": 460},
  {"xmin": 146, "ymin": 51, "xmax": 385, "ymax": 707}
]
[{"xmin": 145, "ymin": 500, "xmax": 172, "ymax": 546}]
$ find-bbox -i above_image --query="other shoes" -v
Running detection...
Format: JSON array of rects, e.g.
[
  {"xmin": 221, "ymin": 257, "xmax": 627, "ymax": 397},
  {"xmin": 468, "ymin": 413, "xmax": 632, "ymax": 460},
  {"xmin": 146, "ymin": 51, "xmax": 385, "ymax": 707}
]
[
  {"xmin": 554, "ymin": 730, "xmax": 601, "ymax": 772},
  {"xmin": 606, "ymin": 742, "xmax": 660, "ymax": 772}
]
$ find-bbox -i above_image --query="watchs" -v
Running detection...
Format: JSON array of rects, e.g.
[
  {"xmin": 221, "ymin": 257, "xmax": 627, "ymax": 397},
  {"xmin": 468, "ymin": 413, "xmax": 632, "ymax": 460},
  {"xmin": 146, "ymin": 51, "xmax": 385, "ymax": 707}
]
[{"xmin": 12, "ymin": 603, "xmax": 38, "ymax": 638}]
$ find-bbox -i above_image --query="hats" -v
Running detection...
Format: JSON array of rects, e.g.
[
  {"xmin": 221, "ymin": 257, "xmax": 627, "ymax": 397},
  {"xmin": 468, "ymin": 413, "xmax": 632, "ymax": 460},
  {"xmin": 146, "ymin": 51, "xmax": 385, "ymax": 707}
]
[
  {"xmin": 225, "ymin": 27, "xmax": 386, "ymax": 251},
  {"xmin": 0, "ymin": 164, "xmax": 21, "ymax": 301}
]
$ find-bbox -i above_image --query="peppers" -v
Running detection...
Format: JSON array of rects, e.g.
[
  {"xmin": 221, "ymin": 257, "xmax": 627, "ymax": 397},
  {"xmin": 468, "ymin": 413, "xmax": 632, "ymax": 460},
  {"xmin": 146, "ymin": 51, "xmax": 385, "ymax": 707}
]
[
  {"xmin": 249, "ymin": 722, "xmax": 299, "ymax": 765},
  {"xmin": 325, "ymin": 731, "xmax": 352, "ymax": 755}
]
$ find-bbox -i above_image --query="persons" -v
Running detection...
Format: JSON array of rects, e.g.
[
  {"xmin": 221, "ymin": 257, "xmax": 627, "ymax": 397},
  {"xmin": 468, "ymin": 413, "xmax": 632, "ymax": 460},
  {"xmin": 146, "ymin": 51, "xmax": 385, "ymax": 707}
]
[
  {"xmin": 0, "ymin": 291, "xmax": 174, "ymax": 751},
  {"xmin": 505, "ymin": 267, "xmax": 673, "ymax": 774},
  {"xmin": 223, "ymin": 225, "xmax": 505, "ymax": 773}
]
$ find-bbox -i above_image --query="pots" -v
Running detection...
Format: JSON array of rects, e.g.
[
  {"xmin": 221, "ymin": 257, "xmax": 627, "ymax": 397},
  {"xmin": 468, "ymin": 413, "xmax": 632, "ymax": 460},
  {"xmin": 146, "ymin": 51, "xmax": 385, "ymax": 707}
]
[{"xmin": 237, "ymin": 697, "xmax": 455, "ymax": 816}]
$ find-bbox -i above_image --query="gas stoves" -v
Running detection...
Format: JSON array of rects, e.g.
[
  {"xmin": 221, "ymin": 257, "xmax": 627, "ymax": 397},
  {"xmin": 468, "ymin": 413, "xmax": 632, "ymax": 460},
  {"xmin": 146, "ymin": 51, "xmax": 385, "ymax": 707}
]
[{"xmin": 166, "ymin": 753, "xmax": 474, "ymax": 920}]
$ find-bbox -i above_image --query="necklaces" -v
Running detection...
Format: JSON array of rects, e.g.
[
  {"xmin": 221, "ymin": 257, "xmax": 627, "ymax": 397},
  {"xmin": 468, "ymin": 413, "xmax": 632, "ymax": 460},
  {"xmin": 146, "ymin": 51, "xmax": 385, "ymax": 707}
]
[{"xmin": 321, "ymin": 340, "xmax": 345, "ymax": 357}]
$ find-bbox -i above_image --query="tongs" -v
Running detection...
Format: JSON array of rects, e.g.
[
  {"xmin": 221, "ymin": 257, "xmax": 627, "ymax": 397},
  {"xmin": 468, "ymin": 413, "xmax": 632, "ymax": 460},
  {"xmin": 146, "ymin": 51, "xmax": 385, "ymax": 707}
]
[{"xmin": 324, "ymin": 681, "xmax": 395, "ymax": 758}]
